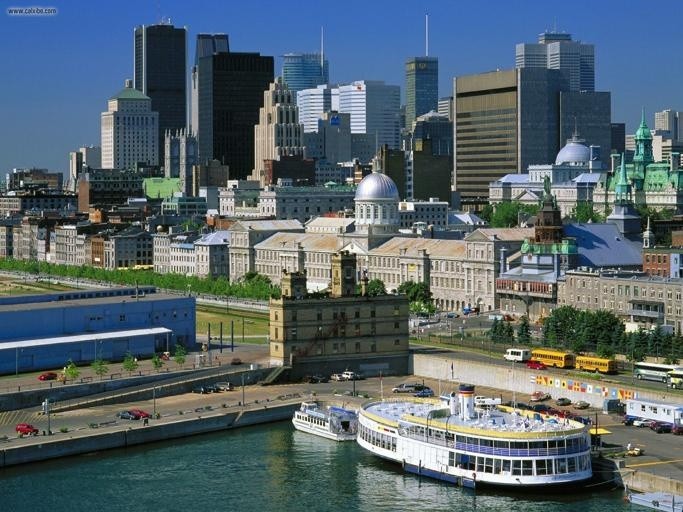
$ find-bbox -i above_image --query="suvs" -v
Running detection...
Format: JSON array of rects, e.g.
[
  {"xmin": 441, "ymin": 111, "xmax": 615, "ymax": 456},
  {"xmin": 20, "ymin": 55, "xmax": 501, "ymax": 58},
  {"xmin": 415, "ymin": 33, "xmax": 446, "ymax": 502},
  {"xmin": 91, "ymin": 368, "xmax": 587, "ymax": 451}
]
[
  {"xmin": 15, "ymin": 423, "xmax": 39, "ymax": 435},
  {"xmin": 573, "ymin": 400, "xmax": 591, "ymax": 410},
  {"xmin": 620, "ymin": 415, "xmax": 683, "ymax": 435},
  {"xmin": 527, "ymin": 359, "xmax": 546, "ymax": 370}
]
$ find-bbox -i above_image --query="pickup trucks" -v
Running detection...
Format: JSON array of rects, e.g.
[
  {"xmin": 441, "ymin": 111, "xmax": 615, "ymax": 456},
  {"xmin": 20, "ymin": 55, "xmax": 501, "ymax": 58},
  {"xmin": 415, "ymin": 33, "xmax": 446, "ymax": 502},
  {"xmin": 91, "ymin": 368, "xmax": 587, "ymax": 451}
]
[{"xmin": 392, "ymin": 384, "xmax": 416, "ymax": 393}]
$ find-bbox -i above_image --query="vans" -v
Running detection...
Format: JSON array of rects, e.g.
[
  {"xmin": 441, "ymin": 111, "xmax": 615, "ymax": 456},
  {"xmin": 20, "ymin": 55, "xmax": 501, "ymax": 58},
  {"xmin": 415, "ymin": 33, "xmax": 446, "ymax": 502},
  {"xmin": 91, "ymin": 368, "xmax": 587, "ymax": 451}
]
[{"xmin": 503, "ymin": 348, "xmax": 532, "ymax": 363}]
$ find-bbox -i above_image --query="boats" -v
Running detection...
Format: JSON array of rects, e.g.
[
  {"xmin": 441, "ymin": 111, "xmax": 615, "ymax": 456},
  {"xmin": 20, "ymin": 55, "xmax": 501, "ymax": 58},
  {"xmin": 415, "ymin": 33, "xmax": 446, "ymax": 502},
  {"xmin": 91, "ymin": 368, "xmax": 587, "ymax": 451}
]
[
  {"xmin": 292, "ymin": 397, "xmax": 358, "ymax": 441},
  {"xmin": 357, "ymin": 383, "xmax": 593, "ymax": 490}
]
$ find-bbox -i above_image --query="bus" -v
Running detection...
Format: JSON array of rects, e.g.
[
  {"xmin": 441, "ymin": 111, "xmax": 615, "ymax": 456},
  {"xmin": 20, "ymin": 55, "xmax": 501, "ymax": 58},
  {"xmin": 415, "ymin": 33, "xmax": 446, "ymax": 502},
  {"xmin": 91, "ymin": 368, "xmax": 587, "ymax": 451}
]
[
  {"xmin": 633, "ymin": 361, "xmax": 683, "ymax": 390},
  {"xmin": 530, "ymin": 349, "xmax": 617, "ymax": 375}
]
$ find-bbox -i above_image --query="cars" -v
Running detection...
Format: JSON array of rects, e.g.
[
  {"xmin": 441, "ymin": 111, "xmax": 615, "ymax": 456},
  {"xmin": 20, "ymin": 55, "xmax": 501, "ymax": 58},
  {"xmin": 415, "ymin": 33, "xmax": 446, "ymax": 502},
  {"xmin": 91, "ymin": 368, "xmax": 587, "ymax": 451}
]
[
  {"xmin": 412, "ymin": 389, "xmax": 434, "ymax": 397},
  {"xmin": 529, "ymin": 391, "xmax": 552, "ymax": 402},
  {"xmin": 230, "ymin": 358, "xmax": 241, "ymax": 364},
  {"xmin": 38, "ymin": 372, "xmax": 57, "ymax": 381},
  {"xmin": 192, "ymin": 382, "xmax": 233, "ymax": 394},
  {"xmin": 444, "ymin": 312, "xmax": 460, "ymax": 318},
  {"xmin": 302, "ymin": 372, "xmax": 365, "ymax": 384},
  {"xmin": 116, "ymin": 409, "xmax": 149, "ymax": 420},
  {"xmin": 462, "ymin": 308, "xmax": 472, "ymax": 315},
  {"xmin": 556, "ymin": 397, "xmax": 572, "ymax": 406}
]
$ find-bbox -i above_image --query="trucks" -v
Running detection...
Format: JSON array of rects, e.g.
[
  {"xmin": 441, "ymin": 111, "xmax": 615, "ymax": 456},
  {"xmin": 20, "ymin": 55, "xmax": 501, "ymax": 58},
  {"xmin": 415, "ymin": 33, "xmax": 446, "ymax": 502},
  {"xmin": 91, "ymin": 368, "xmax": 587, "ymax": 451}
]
[{"xmin": 489, "ymin": 314, "xmax": 509, "ymax": 321}]
